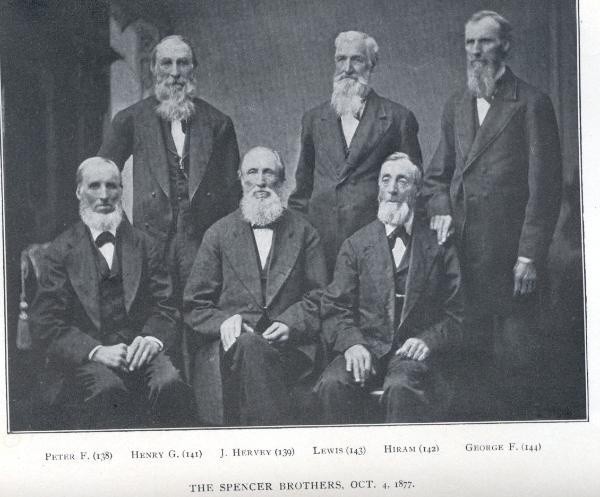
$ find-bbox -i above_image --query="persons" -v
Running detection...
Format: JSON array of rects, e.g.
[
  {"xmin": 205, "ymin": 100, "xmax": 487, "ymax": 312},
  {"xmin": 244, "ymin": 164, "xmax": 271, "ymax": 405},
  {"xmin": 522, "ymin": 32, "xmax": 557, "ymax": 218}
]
[
  {"xmin": 422, "ymin": 8, "xmax": 564, "ymax": 421},
  {"xmin": 287, "ymin": 30, "xmax": 423, "ymax": 283},
  {"xmin": 27, "ymin": 157, "xmax": 195, "ymax": 428},
  {"xmin": 97, "ymin": 32, "xmax": 243, "ymax": 377},
  {"xmin": 182, "ymin": 143, "xmax": 328, "ymax": 427},
  {"xmin": 311, "ymin": 150, "xmax": 472, "ymax": 424}
]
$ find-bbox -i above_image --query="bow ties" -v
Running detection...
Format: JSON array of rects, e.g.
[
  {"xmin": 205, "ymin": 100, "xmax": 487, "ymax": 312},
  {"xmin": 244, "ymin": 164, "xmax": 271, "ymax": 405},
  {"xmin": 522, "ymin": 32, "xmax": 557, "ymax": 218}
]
[
  {"xmin": 388, "ymin": 224, "xmax": 410, "ymax": 249},
  {"xmin": 252, "ymin": 221, "xmax": 273, "ymax": 228},
  {"xmin": 95, "ymin": 230, "xmax": 115, "ymax": 246}
]
